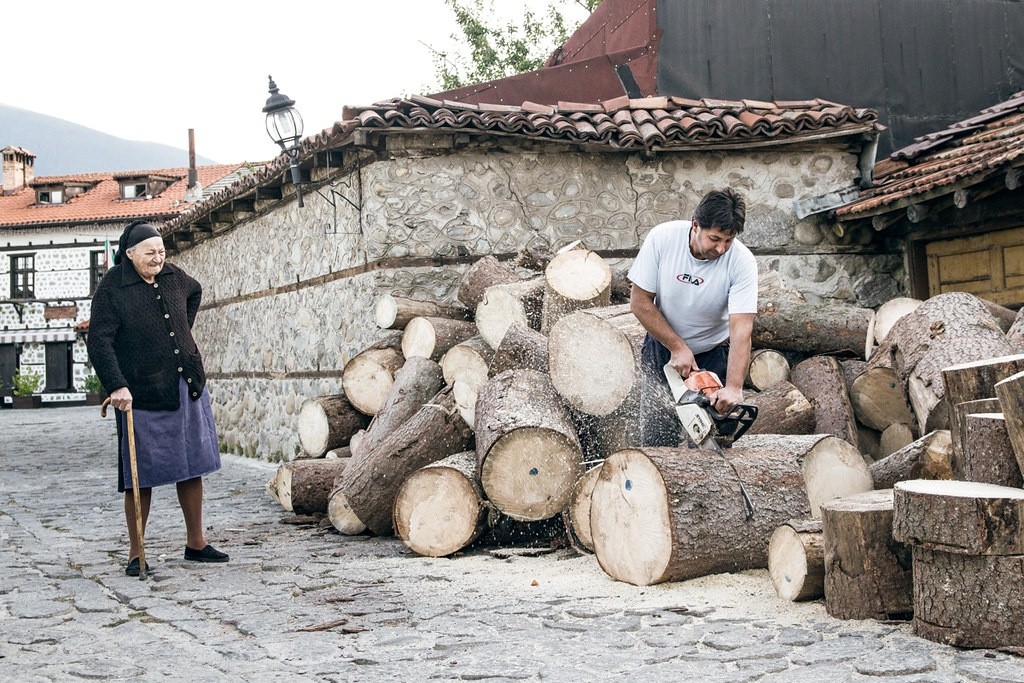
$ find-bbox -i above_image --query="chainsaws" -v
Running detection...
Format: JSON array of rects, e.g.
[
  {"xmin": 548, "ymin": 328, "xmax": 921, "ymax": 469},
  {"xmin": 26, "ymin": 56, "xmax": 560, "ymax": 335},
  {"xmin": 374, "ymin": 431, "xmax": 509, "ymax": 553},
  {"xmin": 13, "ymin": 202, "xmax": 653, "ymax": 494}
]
[{"xmin": 664, "ymin": 363, "xmax": 759, "ymax": 520}]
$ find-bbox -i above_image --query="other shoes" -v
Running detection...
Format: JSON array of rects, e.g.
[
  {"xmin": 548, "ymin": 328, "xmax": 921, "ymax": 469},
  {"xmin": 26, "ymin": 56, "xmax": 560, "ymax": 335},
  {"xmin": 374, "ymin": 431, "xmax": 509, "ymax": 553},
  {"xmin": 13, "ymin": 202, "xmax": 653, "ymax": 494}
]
[
  {"xmin": 184, "ymin": 545, "xmax": 229, "ymax": 562},
  {"xmin": 126, "ymin": 557, "xmax": 149, "ymax": 576}
]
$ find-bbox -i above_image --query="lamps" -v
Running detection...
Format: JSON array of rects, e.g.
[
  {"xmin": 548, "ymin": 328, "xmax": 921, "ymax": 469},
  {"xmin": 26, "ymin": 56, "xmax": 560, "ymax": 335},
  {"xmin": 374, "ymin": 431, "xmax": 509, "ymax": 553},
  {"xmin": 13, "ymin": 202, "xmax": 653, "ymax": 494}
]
[{"xmin": 263, "ymin": 76, "xmax": 364, "ymax": 236}]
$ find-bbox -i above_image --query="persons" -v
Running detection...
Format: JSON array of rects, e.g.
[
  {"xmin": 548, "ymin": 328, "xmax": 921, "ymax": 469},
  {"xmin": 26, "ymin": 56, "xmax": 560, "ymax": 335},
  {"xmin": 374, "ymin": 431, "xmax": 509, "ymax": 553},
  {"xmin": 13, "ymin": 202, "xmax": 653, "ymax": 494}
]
[
  {"xmin": 88, "ymin": 221, "xmax": 229, "ymax": 576},
  {"xmin": 627, "ymin": 187, "xmax": 759, "ymax": 447}
]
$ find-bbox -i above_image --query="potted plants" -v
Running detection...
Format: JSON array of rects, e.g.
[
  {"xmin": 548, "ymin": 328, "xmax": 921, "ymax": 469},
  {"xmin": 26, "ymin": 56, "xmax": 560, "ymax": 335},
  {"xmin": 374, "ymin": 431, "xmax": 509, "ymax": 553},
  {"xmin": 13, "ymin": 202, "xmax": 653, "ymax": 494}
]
[
  {"xmin": 9, "ymin": 368, "xmax": 44, "ymax": 408},
  {"xmin": 85, "ymin": 375, "xmax": 103, "ymax": 405}
]
